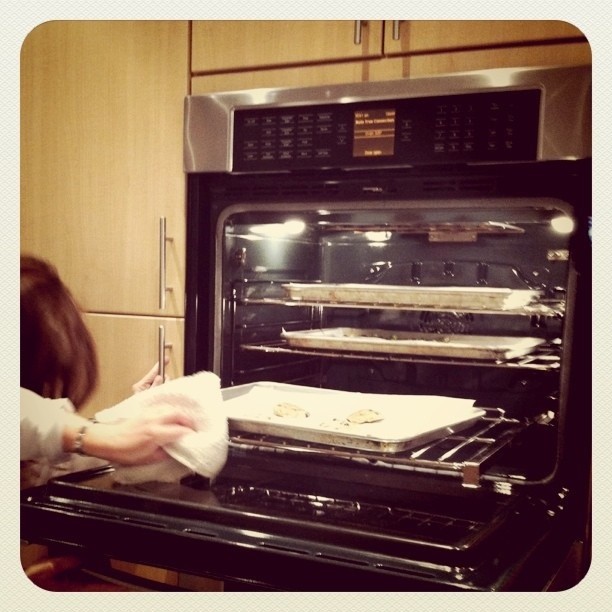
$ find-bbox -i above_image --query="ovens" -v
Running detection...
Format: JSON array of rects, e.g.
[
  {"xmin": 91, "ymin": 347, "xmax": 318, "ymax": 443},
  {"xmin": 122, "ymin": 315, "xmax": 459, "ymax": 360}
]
[{"xmin": 20, "ymin": 63, "xmax": 592, "ymax": 593}]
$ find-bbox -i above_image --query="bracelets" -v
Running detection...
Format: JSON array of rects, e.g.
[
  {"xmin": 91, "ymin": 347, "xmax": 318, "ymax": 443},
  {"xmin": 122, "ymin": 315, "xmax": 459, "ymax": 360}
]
[{"xmin": 71, "ymin": 416, "xmax": 99, "ymax": 454}]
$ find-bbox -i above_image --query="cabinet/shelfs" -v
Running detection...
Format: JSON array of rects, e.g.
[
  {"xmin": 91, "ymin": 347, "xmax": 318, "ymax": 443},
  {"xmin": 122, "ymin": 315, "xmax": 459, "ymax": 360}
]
[
  {"xmin": 189, "ymin": 22, "xmax": 593, "ymax": 93},
  {"xmin": 19, "ymin": 20, "xmax": 191, "ymax": 474}
]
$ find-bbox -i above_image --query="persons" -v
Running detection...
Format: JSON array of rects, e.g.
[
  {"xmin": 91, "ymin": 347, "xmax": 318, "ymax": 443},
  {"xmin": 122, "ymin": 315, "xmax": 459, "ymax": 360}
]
[
  {"xmin": 21, "ymin": 254, "xmax": 210, "ymax": 466},
  {"xmin": 130, "ymin": 355, "xmax": 172, "ymax": 395}
]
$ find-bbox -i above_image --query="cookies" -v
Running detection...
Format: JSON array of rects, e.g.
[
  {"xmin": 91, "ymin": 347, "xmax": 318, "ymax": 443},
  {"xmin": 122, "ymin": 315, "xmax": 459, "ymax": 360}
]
[
  {"xmin": 345, "ymin": 407, "xmax": 385, "ymax": 423},
  {"xmin": 273, "ymin": 401, "xmax": 310, "ymax": 419}
]
